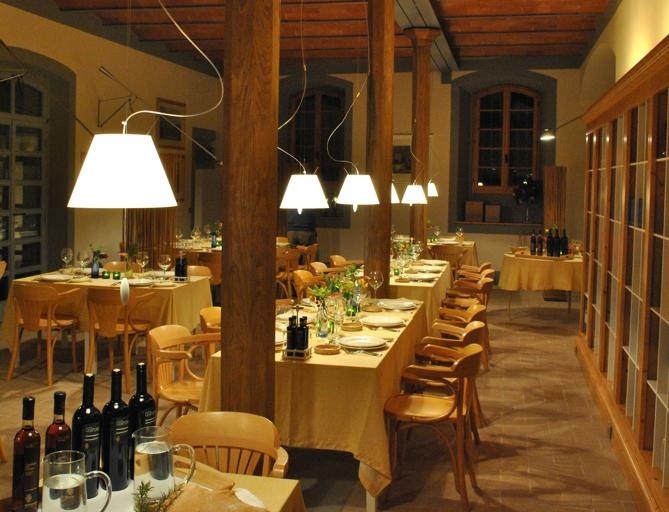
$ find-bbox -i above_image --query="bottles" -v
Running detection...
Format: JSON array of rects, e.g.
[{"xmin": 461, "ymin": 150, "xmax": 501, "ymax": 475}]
[
  {"xmin": 210, "ymin": 232, "xmax": 218, "ymax": 248},
  {"xmin": 100, "ymin": 366, "xmax": 133, "ymax": 493},
  {"xmin": 11, "ymin": 394, "xmax": 40, "ymax": 512},
  {"xmin": 44, "ymin": 390, "xmax": 74, "ymax": 452},
  {"xmin": 78, "ymin": 371, "xmax": 104, "ymax": 499},
  {"xmin": 527, "ymin": 226, "xmax": 570, "ymax": 258},
  {"xmin": 90, "ymin": 252, "xmax": 99, "ymax": 279},
  {"xmin": 128, "ymin": 362, "xmax": 164, "ymax": 444},
  {"xmin": 287, "ymin": 315, "xmax": 309, "ymax": 360}
]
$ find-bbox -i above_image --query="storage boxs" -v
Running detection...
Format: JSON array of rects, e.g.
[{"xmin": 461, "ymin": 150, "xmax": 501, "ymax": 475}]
[
  {"xmin": 485, "ymin": 205, "xmax": 501, "ymax": 223},
  {"xmin": 465, "ymin": 200, "xmax": 484, "ymax": 222}
]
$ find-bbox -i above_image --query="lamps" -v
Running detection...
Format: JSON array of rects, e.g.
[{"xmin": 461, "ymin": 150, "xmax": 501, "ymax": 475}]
[
  {"xmin": 428, "ymin": 165, "xmax": 439, "ymax": 197},
  {"xmin": 277, "ymin": 1, "xmax": 330, "ymax": 215},
  {"xmin": 65, "ymin": 0, "xmax": 225, "ymax": 209},
  {"xmin": 401, "ymin": 135, "xmax": 428, "ymax": 207},
  {"xmin": 540, "ymin": 114, "xmax": 581, "ymax": 141},
  {"xmin": 391, "ymin": 183, "xmax": 400, "ymax": 204},
  {"xmin": 327, "ymin": 0, "xmax": 380, "ymax": 213}
]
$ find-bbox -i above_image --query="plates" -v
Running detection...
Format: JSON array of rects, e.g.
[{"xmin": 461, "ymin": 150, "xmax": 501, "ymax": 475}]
[
  {"xmin": 276, "ymin": 294, "xmax": 324, "ymax": 347},
  {"xmin": 122, "ymin": 278, "xmax": 153, "ymax": 286},
  {"xmin": 360, "ymin": 297, "xmax": 415, "ymax": 328},
  {"xmin": 40, "ymin": 275, "xmax": 74, "ymax": 282},
  {"xmin": 339, "ymin": 337, "xmax": 387, "ymax": 351}
]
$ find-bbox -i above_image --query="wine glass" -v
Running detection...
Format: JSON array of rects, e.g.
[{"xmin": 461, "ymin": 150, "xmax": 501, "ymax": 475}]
[
  {"xmin": 76, "ymin": 250, "xmax": 91, "ymax": 272},
  {"xmin": 395, "ymin": 242, "xmax": 421, "ymax": 279},
  {"xmin": 136, "ymin": 252, "xmax": 150, "ymax": 274},
  {"xmin": 157, "ymin": 253, "xmax": 173, "ymax": 277},
  {"xmin": 60, "ymin": 248, "xmax": 75, "ymax": 270},
  {"xmin": 324, "ymin": 267, "xmax": 385, "ymax": 346},
  {"xmin": 433, "ymin": 226, "xmax": 466, "ymax": 243},
  {"xmin": 175, "ymin": 222, "xmax": 222, "ymax": 247}
]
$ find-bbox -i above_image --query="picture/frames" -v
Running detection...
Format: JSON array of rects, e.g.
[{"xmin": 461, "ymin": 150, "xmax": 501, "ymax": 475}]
[
  {"xmin": 392, "ymin": 134, "xmax": 432, "ymax": 183},
  {"xmin": 156, "ymin": 97, "xmax": 186, "ymax": 150}
]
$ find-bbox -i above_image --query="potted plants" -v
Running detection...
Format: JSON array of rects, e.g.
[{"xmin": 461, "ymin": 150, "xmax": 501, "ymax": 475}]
[{"xmin": 513, "ymin": 173, "xmax": 544, "ymax": 223}]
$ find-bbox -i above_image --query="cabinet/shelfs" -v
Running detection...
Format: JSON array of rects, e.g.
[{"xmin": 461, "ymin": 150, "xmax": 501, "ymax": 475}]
[{"xmin": 0, "ymin": 74, "xmax": 51, "ymax": 302}]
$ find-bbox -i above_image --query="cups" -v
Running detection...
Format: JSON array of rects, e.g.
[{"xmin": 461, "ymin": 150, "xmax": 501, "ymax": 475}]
[
  {"xmin": 128, "ymin": 423, "xmax": 198, "ymax": 507},
  {"xmin": 174, "ymin": 251, "xmax": 189, "ymax": 282},
  {"xmin": 36, "ymin": 450, "xmax": 112, "ymax": 511}
]
